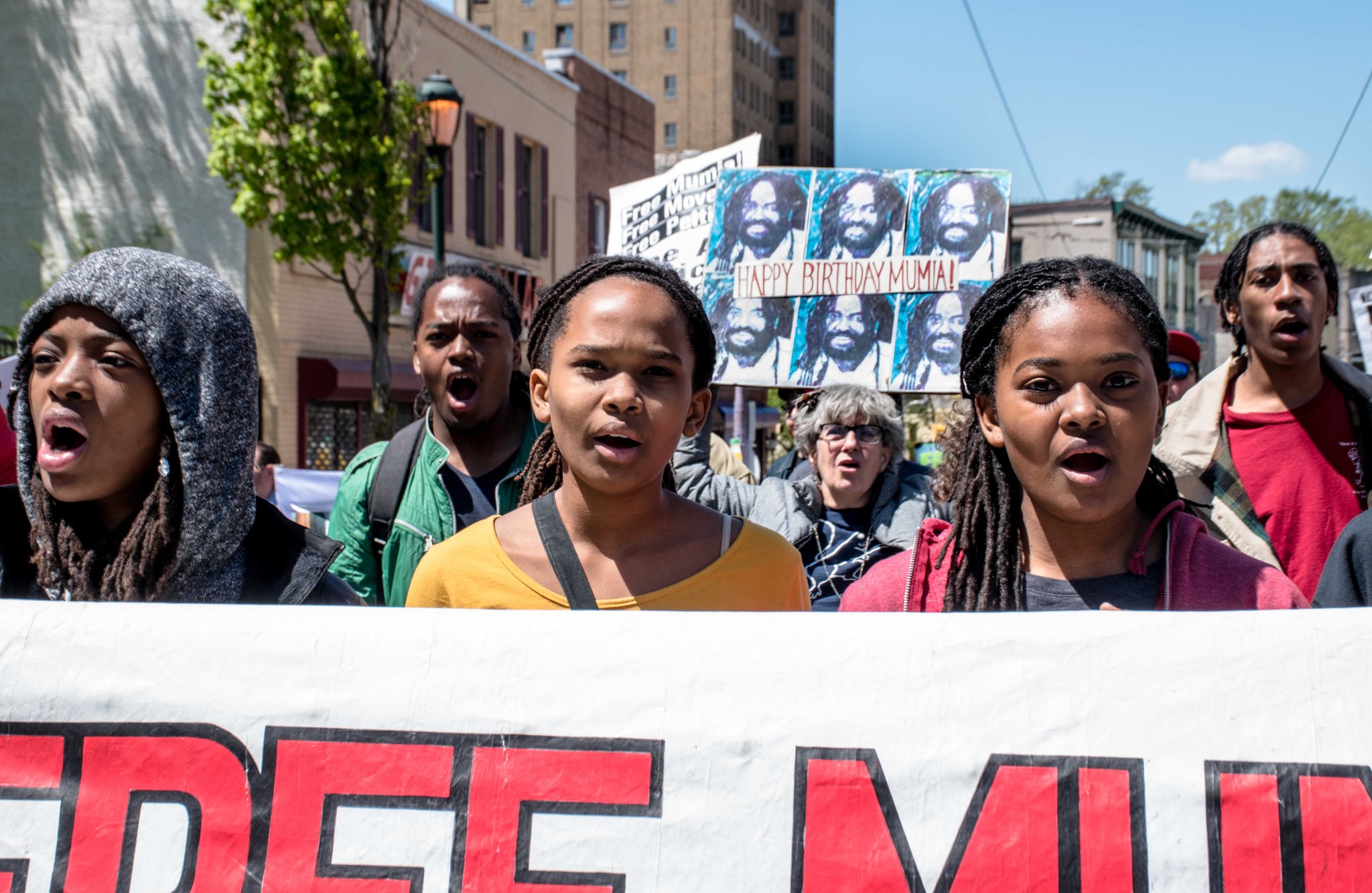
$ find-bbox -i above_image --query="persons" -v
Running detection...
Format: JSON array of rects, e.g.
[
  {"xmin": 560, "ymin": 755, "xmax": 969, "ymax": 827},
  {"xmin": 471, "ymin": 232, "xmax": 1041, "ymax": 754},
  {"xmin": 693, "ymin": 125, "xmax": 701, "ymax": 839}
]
[
  {"xmin": 403, "ymin": 253, "xmax": 812, "ymax": 611},
  {"xmin": 668, "ymin": 383, "xmax": 960, "ymax": 613},
  {"xmin": 836, "ymin": 255, "xmax": 1313, "ymax": 613},
  {"xmin": 1148, "ymin": 220, "xmax": 1372, "ymax": 604},
  {"xmin": 0, "ymin": 246, "xmax": 366, "ymax": 606},
  {"xmin": 1165, "ymin": 329, "xmax": 1201, "ymax": 406},
  {"xmin": 327, "ymin": 262, "xmax": 554, "ymax": 607},
  {"xmin": 706, "ymin": 170, "xmax": 1006, "ymax": 392},
  {"xmin": 1311, "ymin": 512, "xmax": 1372, "ymax": 608}
]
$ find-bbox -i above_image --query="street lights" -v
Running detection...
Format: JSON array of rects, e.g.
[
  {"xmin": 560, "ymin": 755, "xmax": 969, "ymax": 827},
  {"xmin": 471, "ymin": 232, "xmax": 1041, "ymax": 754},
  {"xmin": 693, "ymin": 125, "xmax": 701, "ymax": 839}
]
[{"xmin": 410, "ymin": 67, "xmax": 467, "ymax": 278}]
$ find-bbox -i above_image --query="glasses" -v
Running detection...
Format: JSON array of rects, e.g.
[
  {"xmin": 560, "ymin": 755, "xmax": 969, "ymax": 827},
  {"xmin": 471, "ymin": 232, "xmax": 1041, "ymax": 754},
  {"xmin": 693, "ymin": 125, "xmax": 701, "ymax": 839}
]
[
  {"xmin": 1167, "ymin": 331, "xmax": 1201, "ymax": 363},
  {"xmin": 814, "ymin": 423, "xmax": 886, "ymax": 444},
  {"xmin": 1167, "ymin": 360, "xmax": 1194, "ymax": 381}
]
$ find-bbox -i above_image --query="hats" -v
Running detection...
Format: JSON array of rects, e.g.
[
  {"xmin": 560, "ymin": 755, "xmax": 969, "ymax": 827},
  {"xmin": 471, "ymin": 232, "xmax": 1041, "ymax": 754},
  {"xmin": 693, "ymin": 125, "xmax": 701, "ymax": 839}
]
[{"xmin": 777, "ymin": 387, "xmax": 818, "ymax": 412}]
